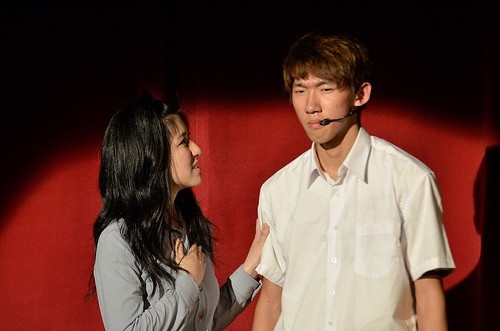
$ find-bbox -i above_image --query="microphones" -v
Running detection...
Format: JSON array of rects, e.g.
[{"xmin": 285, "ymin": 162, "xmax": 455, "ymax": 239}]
[{"xmin": 320, "ymin": 105, "xmax": 368, "ymax": 125}]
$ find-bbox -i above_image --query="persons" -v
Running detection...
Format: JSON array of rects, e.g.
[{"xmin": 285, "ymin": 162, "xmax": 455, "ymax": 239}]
[
  {"xmin": 81, "ymin": 98, "xmax": 270, "ymax": 331},
  {"xmin": 251, "ymin": 36, "xmax": 457, "ymax": 331}
]
